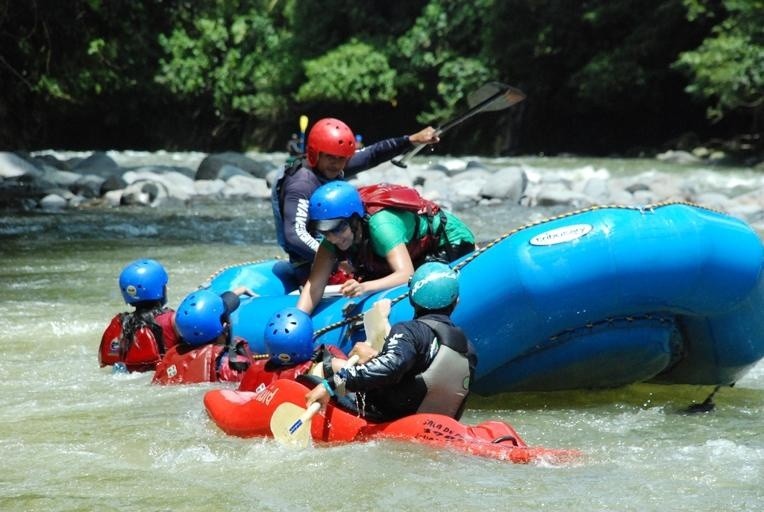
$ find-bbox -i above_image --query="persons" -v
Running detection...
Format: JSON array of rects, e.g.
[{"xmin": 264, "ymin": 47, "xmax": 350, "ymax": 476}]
[
  {"xmin": 282, "ymin": 117, "xmax": 441, "ymax": 259},
  {"xmin": 97, "ymin": 259, "xmax": 260, "ymax": 370},
  {"xmin": 295, "ymin": 179, "xmax": 476, "ymax": 313},
  {"xmin": 305, "ymin": 262, "xmax": 479, "ymax": 424},
  {"xmin": 150, "ymin": 289, "xmax": 252, "ymax": 389}
]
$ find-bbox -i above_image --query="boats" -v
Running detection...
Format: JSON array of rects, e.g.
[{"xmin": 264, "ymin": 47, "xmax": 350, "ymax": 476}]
[{"xmin": 202, "ymin": 377, "xmax": 586, "ymax": 465}]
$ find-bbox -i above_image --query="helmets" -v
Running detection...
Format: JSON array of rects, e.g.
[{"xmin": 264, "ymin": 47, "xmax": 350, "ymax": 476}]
[
  {"xmin": 306, "ymin": 180, "xmax": 366, "ymax": 232},
  {"xmin": 408, "ymin": 261, "xmax": 460, "ymax": 310},
  {"xmin": 263, "ymin": 305, "xmax": 315, "ymax": 365},
  {"xmin": 119, "ymin": 259, "xmax": 168, "ymax": 304},
  {"xmin": 306, "ymin": 118, "xmax": 357, "ymax": 168},
  {"xmin": 174, "ymin": 290, "xmax": 229, "ymax": 346}
]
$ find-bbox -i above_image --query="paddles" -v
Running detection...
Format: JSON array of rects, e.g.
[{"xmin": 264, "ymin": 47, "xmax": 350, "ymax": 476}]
[
  {"xmin": 271, "ymin": 307, "xmax": 391, "ymax": 449},
  {"xmin": 391, "ymin": 81, "xmax": 527, "ymax": 168},
  {"xmin": 299, "ymin": 115, "xmax": 309, "ymax": 152}
]
